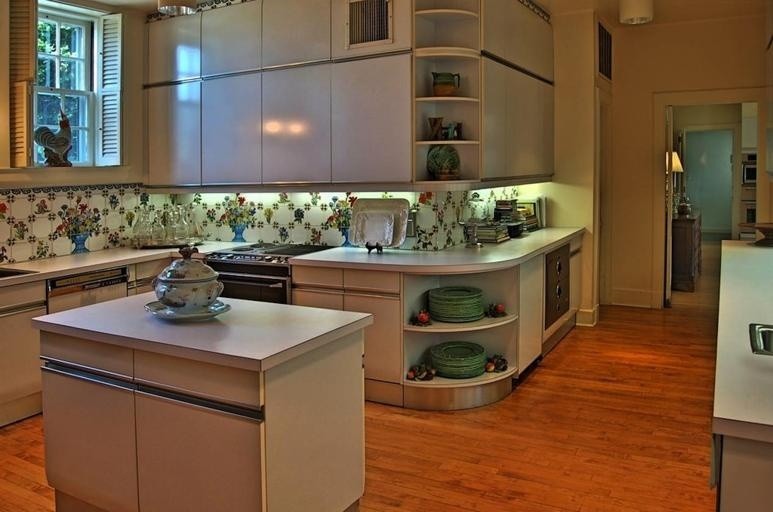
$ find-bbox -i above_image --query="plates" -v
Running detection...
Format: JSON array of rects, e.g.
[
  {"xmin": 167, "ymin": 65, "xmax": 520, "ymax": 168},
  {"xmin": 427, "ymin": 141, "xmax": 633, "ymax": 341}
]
[
  {"xmin": 346, "ymin": 198, "xmax": 409, "ymax": 248},
  {"xmin": 143, "ymin": 300, "xmax": 232, "ymax": 322},
  {"xmin": 352, "ymin": 212, "xmax": 393, "ymax": 245},
  {"xmin": 428, "ymin": 286, "xmax": 485, "ymax": 323},
  {"xmin": 430, "ymin": 341, "xmax": 486, "ymax": 378}
]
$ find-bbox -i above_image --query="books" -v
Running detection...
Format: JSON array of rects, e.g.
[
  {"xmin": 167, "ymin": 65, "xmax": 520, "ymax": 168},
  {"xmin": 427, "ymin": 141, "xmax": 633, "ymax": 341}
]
[{"xmin": 474, "ymin": 199, "xmax": 540, "ymax": 243}]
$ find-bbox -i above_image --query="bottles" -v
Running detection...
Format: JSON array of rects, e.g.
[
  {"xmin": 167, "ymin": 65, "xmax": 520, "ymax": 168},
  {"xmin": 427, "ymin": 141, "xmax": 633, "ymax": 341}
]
[{"xmin": 429, "ymin": 116, "xmax": 461, "ymax": 140}]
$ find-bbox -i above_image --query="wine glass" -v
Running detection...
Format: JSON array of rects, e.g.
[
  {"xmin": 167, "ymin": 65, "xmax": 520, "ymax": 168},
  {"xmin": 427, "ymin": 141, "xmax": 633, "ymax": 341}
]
[{"xmin": 132, "ymin": 202, "xmax": 202, "ymax": 238}]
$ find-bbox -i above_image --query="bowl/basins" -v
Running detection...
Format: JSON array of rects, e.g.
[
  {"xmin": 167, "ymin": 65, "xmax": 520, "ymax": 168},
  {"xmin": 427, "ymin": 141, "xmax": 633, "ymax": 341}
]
[{"xmin": 152, "ymin": 248, "xmax": 218, "ymax": 313}]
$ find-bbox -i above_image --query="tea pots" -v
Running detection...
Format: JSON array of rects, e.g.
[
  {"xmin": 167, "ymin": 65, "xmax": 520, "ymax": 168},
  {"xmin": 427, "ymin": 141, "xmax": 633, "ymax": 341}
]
[{"xmin": 430, "ymin": 70, "xmax": 460, "ymax": 97}]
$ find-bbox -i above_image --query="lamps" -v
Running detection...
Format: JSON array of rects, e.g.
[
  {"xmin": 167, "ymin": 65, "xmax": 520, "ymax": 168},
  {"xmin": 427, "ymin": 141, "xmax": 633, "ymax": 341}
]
[
  {"xmin": 664, "ymin": 149, "xmax": 684, "ymax": 212},
  {"xmin": 616, "ymin": 0, "xmax": 654, "ymax": 26}
]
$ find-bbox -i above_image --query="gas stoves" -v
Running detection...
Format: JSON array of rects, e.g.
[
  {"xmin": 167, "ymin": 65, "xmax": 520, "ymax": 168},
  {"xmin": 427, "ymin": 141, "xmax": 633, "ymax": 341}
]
[{"xmin": 204, "ymin": 241, "xmax": 337, "ymax": 277}]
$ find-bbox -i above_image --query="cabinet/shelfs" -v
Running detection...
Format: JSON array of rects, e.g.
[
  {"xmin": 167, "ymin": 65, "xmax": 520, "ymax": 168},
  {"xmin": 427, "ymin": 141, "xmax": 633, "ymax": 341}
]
[
  {"xmin": 0, "ymin": 279, "xmax": 51, "ymax": 433},
  {"xmin": 262, "ymin": 0, "xmax": 413, "ymax": 184},
  {"xmin": 38, "ymin": 310, "xmax": 368, "ymax": 511},
  {"xmin": 146, "ymin": 0, "xmax": 262, "ymax": 186},
  {"xmin": 517, "ymin": 250, "xmax": 546, "ymax": 386},
  {"xmin": 569, "ymin": 235, "xmax": 584, "ymax": 310},
  {"xmin": 401, "ymin": 267, "xmax": 519, "ymax": 389},
  {"xmin": 480, "ymin": 1, "xmax": 561, "ymax": 188},
  {"xmin": 126, "ymin": 256, "xmax": 173, "ymax": 295},
  {"xmin": 739, "ymin": 102, "xmax": 759, "ymax": 154},
  {"xmin": 290, "ymin": 266, "xmax": 405, "ymax": 408},
  {"xmin": 408, "ymin": 1, "xmax": 478, "ymax": 191},
  {"xmin": 669, "ymin": 204, "xmax": 704, "ymax": 293}
]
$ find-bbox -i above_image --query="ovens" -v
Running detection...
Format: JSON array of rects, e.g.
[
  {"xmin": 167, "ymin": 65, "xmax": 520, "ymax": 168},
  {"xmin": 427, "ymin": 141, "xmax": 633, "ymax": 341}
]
[{"xmin": 215, "ymin": 273, "xmax": 291, "ymax": 305}]
